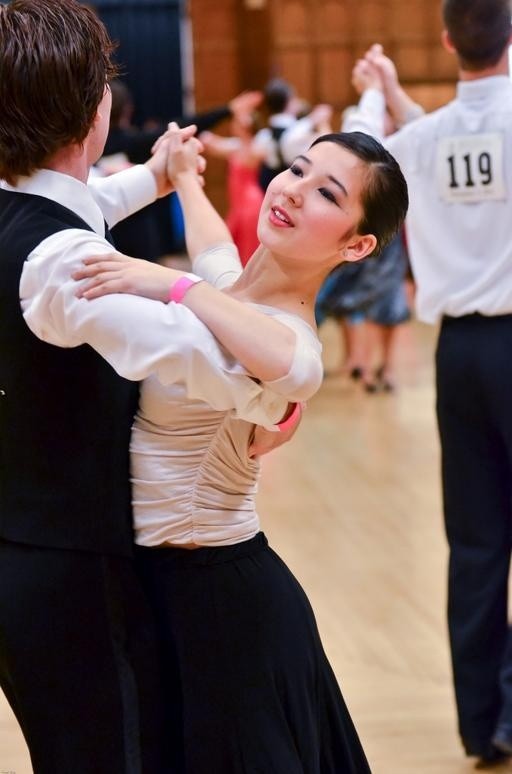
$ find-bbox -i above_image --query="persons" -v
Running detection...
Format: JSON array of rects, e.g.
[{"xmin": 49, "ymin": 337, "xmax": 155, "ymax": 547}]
[
  {"xmin": 0, "ymin": 0, "xmax": 307, "ymax": 772},
  {"xmin": 358, "ymin": 39, "xmax": 426, "ymax": 131},
  {"xmin": 349, "ymin": 0, "xmax": 512, "ymax": 767},
  {"xmin": 70, "ymin": 120, "xmax": 410, "ymax": 772}
]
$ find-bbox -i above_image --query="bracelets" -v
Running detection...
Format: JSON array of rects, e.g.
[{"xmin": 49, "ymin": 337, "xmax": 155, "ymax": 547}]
[{"xmin": 257, "ymin": 401, "xmax": 304, "ymax": 433}]
